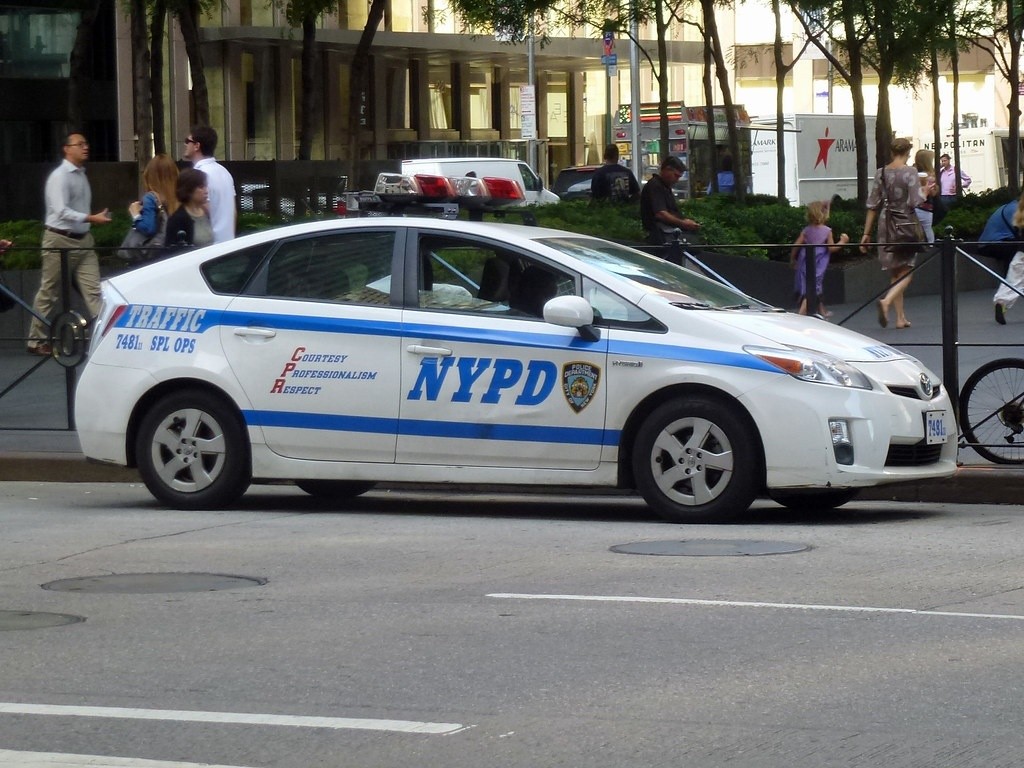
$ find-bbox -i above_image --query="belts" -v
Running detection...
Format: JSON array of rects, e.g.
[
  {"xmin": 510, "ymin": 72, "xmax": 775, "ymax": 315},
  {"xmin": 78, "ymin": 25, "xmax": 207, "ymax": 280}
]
[{"xmin": 44, "ymin": 225, "xmax": 86, "ymax": 240}]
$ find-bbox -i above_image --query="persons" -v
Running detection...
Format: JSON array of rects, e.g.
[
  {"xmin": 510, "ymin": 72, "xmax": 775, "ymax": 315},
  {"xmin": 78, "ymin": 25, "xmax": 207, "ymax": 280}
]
[
  {"xmin": 856, "ymin": 138, "xmax": 1024, "ymax": 329},
  {"xmin": 0, "ymin": 124, "xmax": 236, "ymax": 356},
  {"xmin": 639, "ymin": 157, "xmax": 699, "ymax": 267},
  {"xmin": 589, "ymin": 144, "xmax": 643, "ymax": 203},
  {"xmin": 790, "ymin": 201, "xmax": 849, "ymax": 319}
]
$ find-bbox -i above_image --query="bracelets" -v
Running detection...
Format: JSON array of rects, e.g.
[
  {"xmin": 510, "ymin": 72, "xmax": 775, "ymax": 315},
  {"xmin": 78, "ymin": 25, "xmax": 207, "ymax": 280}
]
[{"xmin": 864, "ymin": 233, "xmax": 871, "ymax": 236}]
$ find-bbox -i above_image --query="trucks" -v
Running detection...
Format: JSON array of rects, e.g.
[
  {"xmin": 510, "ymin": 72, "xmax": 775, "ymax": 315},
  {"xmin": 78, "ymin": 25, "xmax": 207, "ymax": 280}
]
[
  {"xmin": 751, "ymin": 113, "xmax": 877, "ymax": 206},
  {"xmin": 918, "ymin": 128, "xmax": 1024, "ymax": 194}
]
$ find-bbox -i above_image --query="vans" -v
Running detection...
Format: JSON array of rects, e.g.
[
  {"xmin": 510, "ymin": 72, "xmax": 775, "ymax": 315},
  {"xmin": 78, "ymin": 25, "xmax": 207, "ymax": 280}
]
[{"xmin": 401, "ymin": 156, "xmax": 562, "ymax": 207}]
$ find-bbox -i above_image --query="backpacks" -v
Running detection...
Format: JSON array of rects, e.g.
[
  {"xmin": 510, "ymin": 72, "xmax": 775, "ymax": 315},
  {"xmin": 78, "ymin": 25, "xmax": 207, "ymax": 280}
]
[{"xmin": 597, "ymin": 164, "xmax": 631, "ymax": 202}]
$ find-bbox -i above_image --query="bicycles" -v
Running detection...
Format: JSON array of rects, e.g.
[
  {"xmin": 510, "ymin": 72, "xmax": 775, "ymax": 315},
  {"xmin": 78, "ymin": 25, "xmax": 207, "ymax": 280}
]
[{"xmin": 958, "ymin": 356, "xmax": 1024, "ymax": 464}]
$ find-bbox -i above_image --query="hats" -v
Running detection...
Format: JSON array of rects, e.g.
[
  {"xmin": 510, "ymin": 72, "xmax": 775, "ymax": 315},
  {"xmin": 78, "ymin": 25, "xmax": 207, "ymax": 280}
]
[{"xmin": 664, "ymin": 156, "xmax": 689, "ymax": 173}]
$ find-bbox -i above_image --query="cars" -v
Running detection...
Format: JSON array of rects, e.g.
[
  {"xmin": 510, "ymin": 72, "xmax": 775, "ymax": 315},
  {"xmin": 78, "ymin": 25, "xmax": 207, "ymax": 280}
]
[
  {"xmin": 241, "ymin": 184, "xmax": 387, "ymax": 217},
  {"xmin": 553, "ymin": 164, "xmax": 678, "ymax": 203},
  {"xmin": 73, "ymin": 173, "xmax": 959, "ymax": 522}
]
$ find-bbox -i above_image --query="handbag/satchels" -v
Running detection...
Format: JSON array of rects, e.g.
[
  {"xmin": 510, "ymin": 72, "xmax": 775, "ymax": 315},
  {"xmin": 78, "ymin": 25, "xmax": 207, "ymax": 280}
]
[
  {"xmin": 656, "ymin": 213, "xmax": 702, "ymax": 254},
  {"xmin": 115, "ymin": 191, "xmax": 167, "ymax": 260},
  {"xmin": 883, "ymin": 207, "xmax": 927, "ymax": 254}
]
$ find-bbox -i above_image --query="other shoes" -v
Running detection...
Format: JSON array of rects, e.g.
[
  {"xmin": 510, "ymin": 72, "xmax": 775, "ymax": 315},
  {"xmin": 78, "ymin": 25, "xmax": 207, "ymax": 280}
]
[
  {"xmin": 877, "ymin": 298, "xmax": 889, "ymax": 328},
  {"xmin": 895, "ymin": 319, "xmax": 911, "ymax": 329},
  {"xmin": 27, "ymin": 345, "xmax": 51, "ymax": 354},
  {"xmin": 995, "ymin": 302, "xmax": 1006, "ymax": 325}
]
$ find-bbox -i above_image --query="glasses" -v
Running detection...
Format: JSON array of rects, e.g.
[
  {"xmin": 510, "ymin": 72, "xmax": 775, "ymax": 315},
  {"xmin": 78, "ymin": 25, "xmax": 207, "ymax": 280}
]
[
  {"xmin": 184, "ymin": 138, "xmax": 196, "ymax": 144},
  {"xmin": 65, "ymin": 142, "xmax": 90, "ymax": 148}
]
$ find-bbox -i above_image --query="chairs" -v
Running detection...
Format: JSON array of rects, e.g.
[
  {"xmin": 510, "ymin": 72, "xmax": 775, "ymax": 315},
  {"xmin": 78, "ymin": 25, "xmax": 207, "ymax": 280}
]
[{"xmin": 477, "ymin": 258, "xmax": 558, "ymax": 316}]
